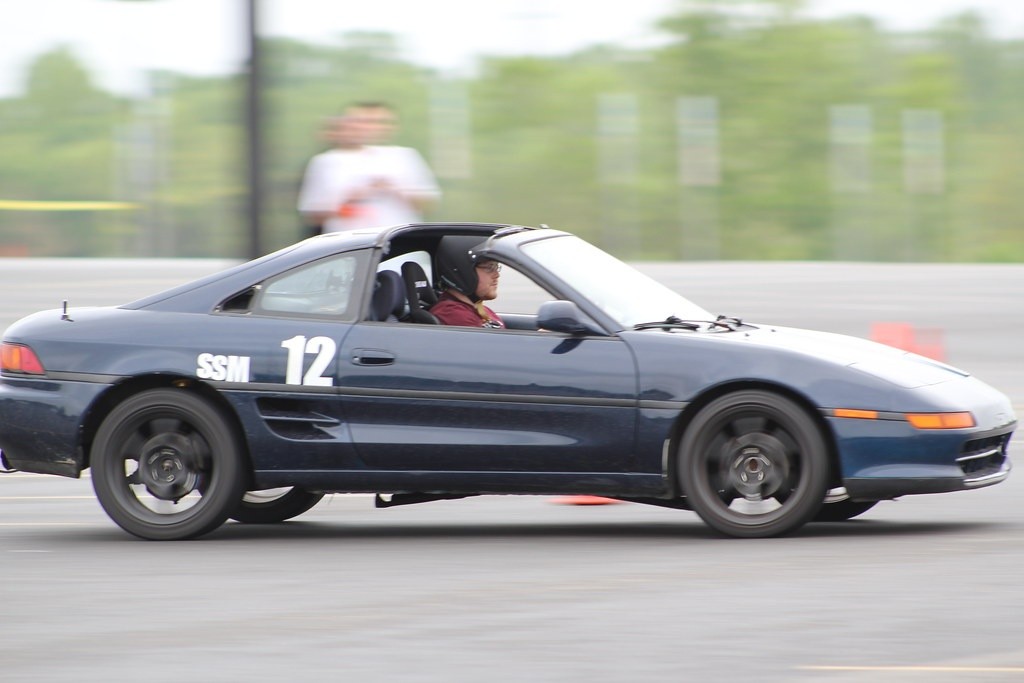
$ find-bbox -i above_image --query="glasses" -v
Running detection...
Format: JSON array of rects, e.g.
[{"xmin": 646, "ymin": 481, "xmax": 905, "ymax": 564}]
[{"xmin": 477, "ymin": 264, "xmax": 501, "ymax": 272}]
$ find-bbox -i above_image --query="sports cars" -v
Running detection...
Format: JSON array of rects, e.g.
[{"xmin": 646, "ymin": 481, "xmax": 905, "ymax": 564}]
[{"xmin": 1, "ymin": 220, "xmax": 1024, "ymax": 540}]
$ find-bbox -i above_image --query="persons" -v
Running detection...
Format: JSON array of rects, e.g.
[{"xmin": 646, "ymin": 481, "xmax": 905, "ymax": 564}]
[
  {"xmin": 296, "ymin": 100, "xmax": 442, "ymax": 234},
  {"xmin": 427, "ymin": 235, "xmax": 550, "ymax": 333}
]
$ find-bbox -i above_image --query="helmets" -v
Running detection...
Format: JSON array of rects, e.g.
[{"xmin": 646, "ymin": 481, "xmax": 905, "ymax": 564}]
[{"xmin": 435, "ymin": 234, "xmax": 493, "ymax": 303}]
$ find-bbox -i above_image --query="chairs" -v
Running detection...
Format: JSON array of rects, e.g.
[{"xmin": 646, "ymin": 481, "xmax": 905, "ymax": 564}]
[
  {"xmin": 402, "ymin": 261, "xmax": 439, "ymax": 324},
  {"xmin": 369, "ymin": 270, "xmax": 406, "ymax": 324}
]
